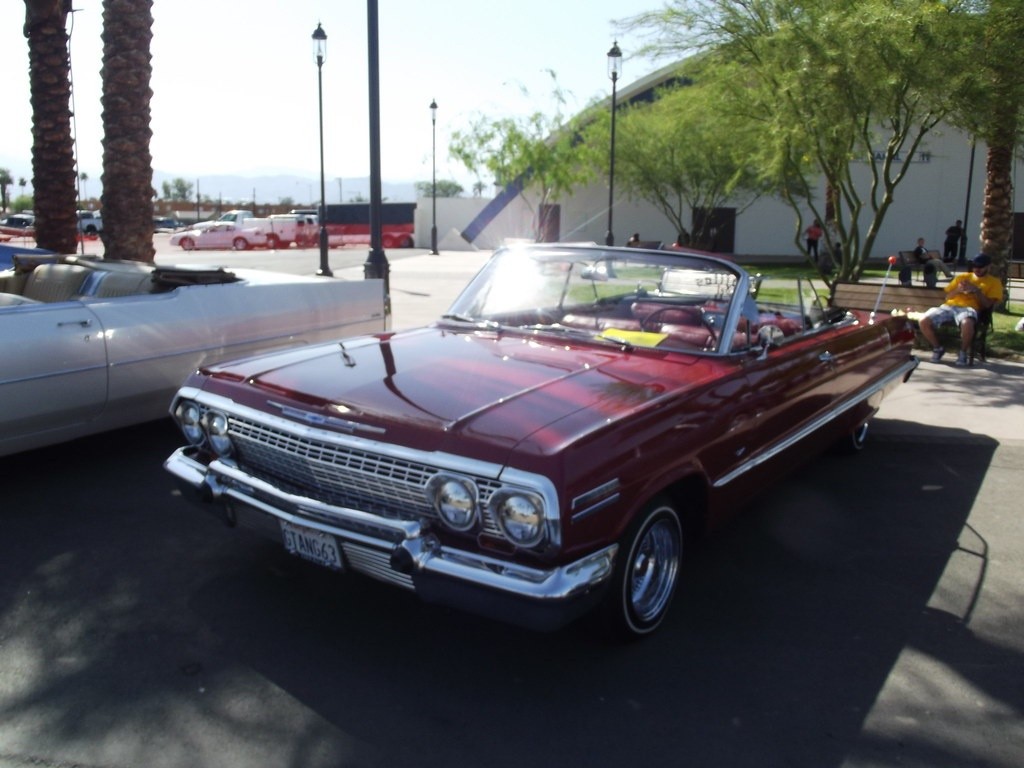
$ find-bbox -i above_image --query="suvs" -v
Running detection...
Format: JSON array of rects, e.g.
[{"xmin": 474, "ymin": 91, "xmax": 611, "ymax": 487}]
[{"xmin": 287, "ymin": 207, "xmax": 320, "ymax": 246}]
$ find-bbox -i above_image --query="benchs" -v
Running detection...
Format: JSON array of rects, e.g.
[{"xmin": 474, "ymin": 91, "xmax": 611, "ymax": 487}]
[
  {"xmin": 628, "ymin": 302, "xmax": 802, "ymax": 336},
  {"xmin": 898, "ymin": 249, "xmax": 957, "ymax": 281},
  {"xmin": 813, "ymin": 282, "xmax": 995, "ymax": 368}
]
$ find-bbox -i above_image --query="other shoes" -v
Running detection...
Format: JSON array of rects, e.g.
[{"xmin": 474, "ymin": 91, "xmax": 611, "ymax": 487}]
[
  {"xmin": 956, "ymin": 349, "xmax": 967, "ymax": 366},
  {"xmin": 931, "ymin": 344, "xmax": 945, "ymax": 362},
  {"xmin": 946, "ymin": 274, "xmax": 954, "ymax": 277}
]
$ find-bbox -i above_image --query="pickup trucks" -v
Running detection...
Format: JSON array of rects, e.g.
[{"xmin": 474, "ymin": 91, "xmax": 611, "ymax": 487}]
[{"xmin": 219, "ymin": 209, "xmax": 298, "ymax": 250}]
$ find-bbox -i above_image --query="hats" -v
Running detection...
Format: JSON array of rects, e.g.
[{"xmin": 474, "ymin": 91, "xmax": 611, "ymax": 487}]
[{"xmin": 972, "ymin": 253, "xmax": 992, "ymax": 267}]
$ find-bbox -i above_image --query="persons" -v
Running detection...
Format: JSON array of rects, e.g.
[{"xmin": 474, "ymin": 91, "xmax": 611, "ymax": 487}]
[
  {"xmin": 627, "ymin": 233, "xmax": 639, "ymax": 246},
  {"xmin": 803, "ymin": 219, "xmax": 822, "ymax": 257},
  {"xmin": 677, "ymin": 229, "xmax": 690, "ymax": 247},
  {"xmin": 943, "ymin": 220, "xmax": 964, "ymax": 262},
  {"xmin": 915, "ymin": 238, "xmax": 954, "ymax": 278},
  {"xmin": 919, "ymin": 253, "xmax": 1004, "ymax": 366}
]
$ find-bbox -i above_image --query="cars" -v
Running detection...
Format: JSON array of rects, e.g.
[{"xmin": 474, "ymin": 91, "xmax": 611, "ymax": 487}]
[
  {"xmin": 169, "ymin": 221, "xmax": 268, "ymax": 251},
  {"xmin": 0, "ymin": 206, "xmax": 185, "ymax": 243}
]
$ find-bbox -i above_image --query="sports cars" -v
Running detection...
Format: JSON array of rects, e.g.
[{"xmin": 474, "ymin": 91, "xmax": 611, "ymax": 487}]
[
  {"xmin": 160, "ymin": 240, "xmax": 924, "ymax": 644},
  {"xmin": 0, "ymin": 251, "xmax": 387, "ymax": 462}
]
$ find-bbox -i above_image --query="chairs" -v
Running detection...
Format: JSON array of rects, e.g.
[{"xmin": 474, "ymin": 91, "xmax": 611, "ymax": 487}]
[
  {"xmin": 94, "ymin": 272, "xmax": 153, "ymax": 298},
  {"xmin": 23, "ymin": 265, "xmax": 87, "ymax": 304},
  {"xmin": 662, "ymin": 324, "xmax": 752, "ymax": 351},
  {"xmin": 560, "ymin": 315, "xmax": 641, "ymax": 333}
]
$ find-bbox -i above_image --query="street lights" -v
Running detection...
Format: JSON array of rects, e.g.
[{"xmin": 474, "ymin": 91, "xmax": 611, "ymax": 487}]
[
  {"xmin": 428, "ymin": 97, "xmax": 442, "ymax": 256},
  {"xmin": 311, "ymin": 20, "xmax": 333, "ymax": 276},
  {"xmin": 605, "ymin": 37, "xmax": 623, "ymax": 275}
]
